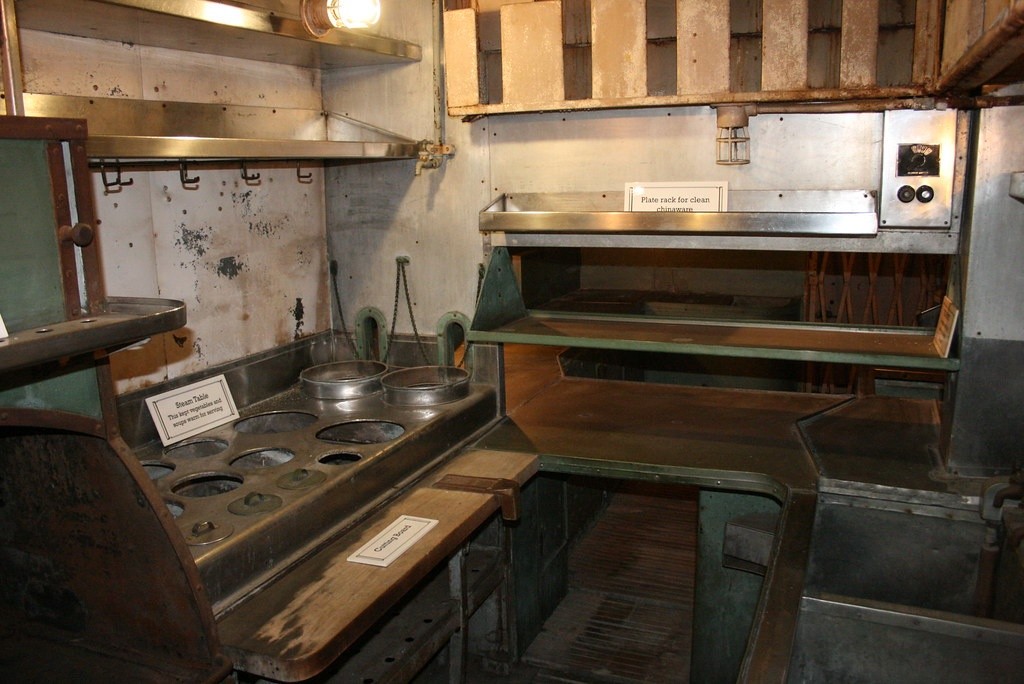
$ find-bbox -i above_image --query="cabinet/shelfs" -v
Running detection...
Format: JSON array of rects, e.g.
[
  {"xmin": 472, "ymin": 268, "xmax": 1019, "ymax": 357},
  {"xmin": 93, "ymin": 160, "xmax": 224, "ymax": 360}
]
[{"xmin": 1, "ymin": 0, "xmax": 423, "ymax": 161}]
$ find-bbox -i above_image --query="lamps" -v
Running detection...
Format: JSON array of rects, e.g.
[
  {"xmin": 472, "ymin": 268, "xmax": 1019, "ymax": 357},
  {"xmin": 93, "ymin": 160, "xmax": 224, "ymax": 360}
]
[{"xmin": 300, "ymin": 0, "xmax": 382, "ymax": 38}]
